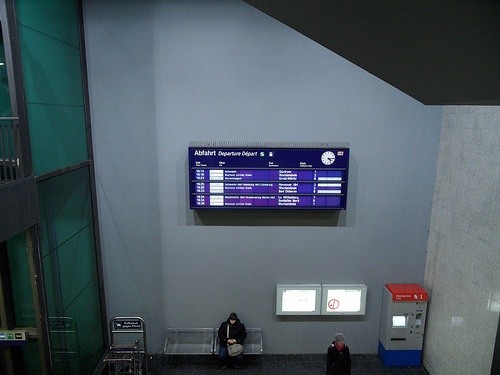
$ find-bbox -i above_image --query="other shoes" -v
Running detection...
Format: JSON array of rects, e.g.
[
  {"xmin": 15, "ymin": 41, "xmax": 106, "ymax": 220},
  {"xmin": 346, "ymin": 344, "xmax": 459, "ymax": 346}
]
[
  {"xmin": 234, "ymin": 362, "xmax": 241, "ymax": 368},
  {"xmin": 220, "ymin": 363, "xmax": 227, "ymax": 369}
]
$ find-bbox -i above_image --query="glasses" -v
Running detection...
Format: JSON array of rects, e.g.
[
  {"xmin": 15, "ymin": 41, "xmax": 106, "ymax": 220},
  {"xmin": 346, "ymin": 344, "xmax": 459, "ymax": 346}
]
[
  {"xmin": 336, "ymin": 342, "xmax": 344, "ymax": 348},
  {"xmin": 229, "ymin": 317, "xmax": 236, "ymax": 320}
]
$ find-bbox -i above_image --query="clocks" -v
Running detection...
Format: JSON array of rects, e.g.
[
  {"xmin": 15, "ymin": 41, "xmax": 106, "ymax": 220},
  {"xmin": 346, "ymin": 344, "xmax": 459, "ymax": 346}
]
[{"xmin": 322, "ymin": 151, "xmax": 336, "ymax": 165}]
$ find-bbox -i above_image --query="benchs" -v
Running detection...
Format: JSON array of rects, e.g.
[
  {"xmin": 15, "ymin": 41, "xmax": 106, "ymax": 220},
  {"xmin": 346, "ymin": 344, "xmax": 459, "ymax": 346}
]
[
  {"xmin": 163, "ymin": 327, "xmax": 216, "ymax": 364},
  {"xmin": 214, "ymin": 327, "xmax": 263, "ymax": 364}
]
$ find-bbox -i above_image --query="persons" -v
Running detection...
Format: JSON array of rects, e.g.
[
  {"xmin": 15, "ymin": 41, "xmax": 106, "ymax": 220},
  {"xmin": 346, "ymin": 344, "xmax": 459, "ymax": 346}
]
[
  {"xmin": 217, "ymin": 312, "xmax": 247, "ymax": 369},
  {"xmin": 326, "ymin": 333, "xmax": 351, "ymax": 375}
]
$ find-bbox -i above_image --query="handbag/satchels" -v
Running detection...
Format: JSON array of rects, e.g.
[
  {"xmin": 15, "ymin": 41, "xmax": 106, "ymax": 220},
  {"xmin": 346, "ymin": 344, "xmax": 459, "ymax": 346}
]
[{"xmin": 228, "ymin": 343, "xmax": 243, "ymax": 356}]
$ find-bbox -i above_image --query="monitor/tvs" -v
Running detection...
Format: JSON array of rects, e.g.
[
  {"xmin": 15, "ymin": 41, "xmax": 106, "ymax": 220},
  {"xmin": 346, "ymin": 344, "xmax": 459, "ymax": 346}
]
[
  {"xmin": 393, "ymin": 315, "xmax": 406, "ymax": 327},
  {"xmin": 321, "ymin": 284, "xmax": 367, "ymax": 316},
  {"xmin": 276, "ymin": 285, "xmax": 321, "ymax": 315}
]
